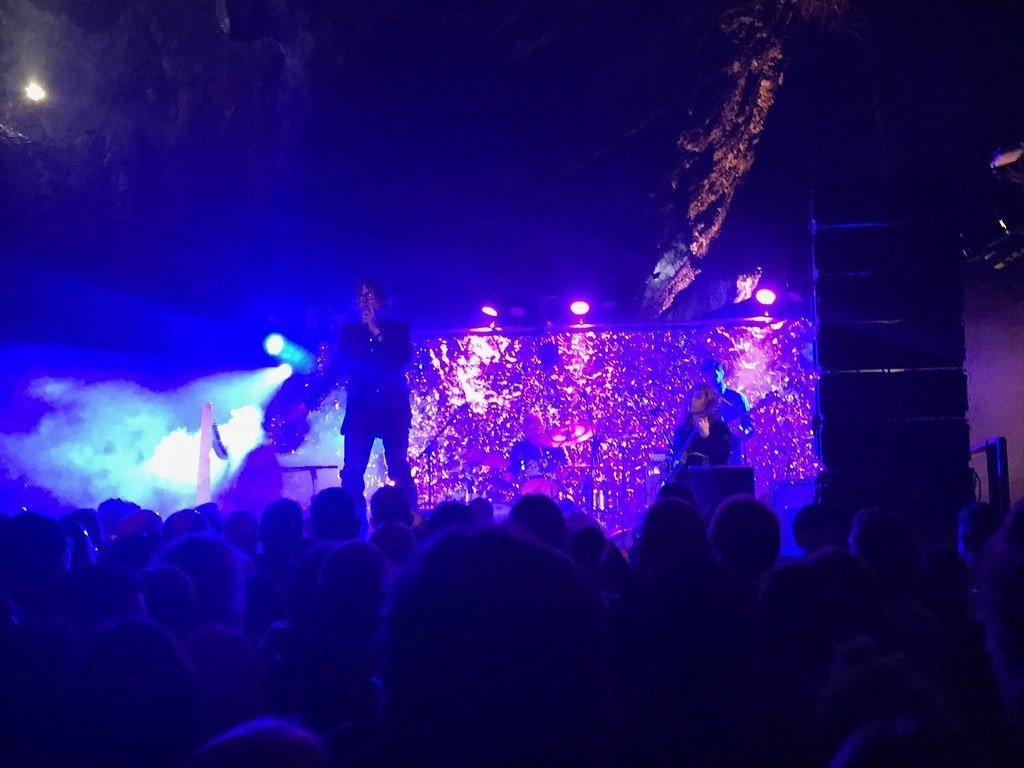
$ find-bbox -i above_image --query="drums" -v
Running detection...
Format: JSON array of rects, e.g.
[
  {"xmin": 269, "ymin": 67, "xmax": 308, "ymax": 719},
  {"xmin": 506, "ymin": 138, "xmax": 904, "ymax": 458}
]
[
  {"xmin": 518, "ymin": 475, "xmax": 566, "ymax": 499},
  {"xmin": 521, "ymin": 453, "xmax": 556, "ymax": 500},
  {"xmin": 479, "ymin": 452, "xmax": 513, "ymax": 488},
  {"xmin": 591, "ymin": 488, "xmax": 620, "ymax": 514}
]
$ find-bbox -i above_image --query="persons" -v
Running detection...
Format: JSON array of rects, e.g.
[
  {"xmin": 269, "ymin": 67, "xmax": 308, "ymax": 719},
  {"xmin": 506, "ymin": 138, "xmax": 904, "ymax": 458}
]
[
  {"xmin": 665, "ymin": 382, "xmax": 734, "ymax": 490},
  {"xmin": 2, "ymin": 487, "xmax": 1024, "ymax": 768},
  {"xmin": 318, "ymin": 275, "xmax": 418, "ymax": 512},
  {"xmin": 702, "ymin": 359, "xmax": 754, "ymax": 466}
]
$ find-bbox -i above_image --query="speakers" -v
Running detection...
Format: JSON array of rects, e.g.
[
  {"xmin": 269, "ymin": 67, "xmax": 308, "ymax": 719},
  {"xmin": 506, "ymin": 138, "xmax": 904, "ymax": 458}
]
[
  {"xmin": 810, "ymin": 211, "xmax": 973, "ymax": 514},
  {"xmin": 683, "ymin": 466, "xmax": 754, "ymax": 527},
  {"xmin": 771, "ymin": 480, "xmax": 822, "ymax": 558}
]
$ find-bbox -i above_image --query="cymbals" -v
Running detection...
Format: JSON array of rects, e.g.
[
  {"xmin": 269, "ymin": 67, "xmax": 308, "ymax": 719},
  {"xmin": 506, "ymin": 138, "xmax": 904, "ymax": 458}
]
[
  {"xmin": 539, "ymin": 425, "xmax": 593, "ymax": 448},
  {"xmin": 566, "ymin": 463, "xmax": 603, "ymax": 470}
]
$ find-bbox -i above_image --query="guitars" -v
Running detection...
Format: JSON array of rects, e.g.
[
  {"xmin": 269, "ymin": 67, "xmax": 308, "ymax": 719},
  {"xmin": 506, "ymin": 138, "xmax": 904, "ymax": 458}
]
[
  {"xmin": 672, "ymin": 400, "xmax": 722, "ymax": 472},
  {"xmin": 727, "ymin": 390, "xmax": 782, "ymax": 429}
]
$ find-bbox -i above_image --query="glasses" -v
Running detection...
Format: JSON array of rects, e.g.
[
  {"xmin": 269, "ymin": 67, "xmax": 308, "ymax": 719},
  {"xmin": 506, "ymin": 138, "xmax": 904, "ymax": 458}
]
[{"xmin": 353, "ymin": 289, "xmax": 377, "ymax": 304}]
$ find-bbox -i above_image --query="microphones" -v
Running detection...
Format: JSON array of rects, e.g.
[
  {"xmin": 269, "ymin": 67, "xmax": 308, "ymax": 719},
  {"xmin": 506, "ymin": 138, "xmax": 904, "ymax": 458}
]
[
  {"xmin": 581, "ymin": 392, "xmax": 587, "ymax": 400},
  {"xmin": 362, "ymin": 306, "xmax": 370, "ymax": 315},
  {"xmin": 649, "ymin": 407, "xmax": 664, "ymax": 413},
  {"xmin": 456, "ymin": 403, "xmax": 470, "ymax": 411}
]
